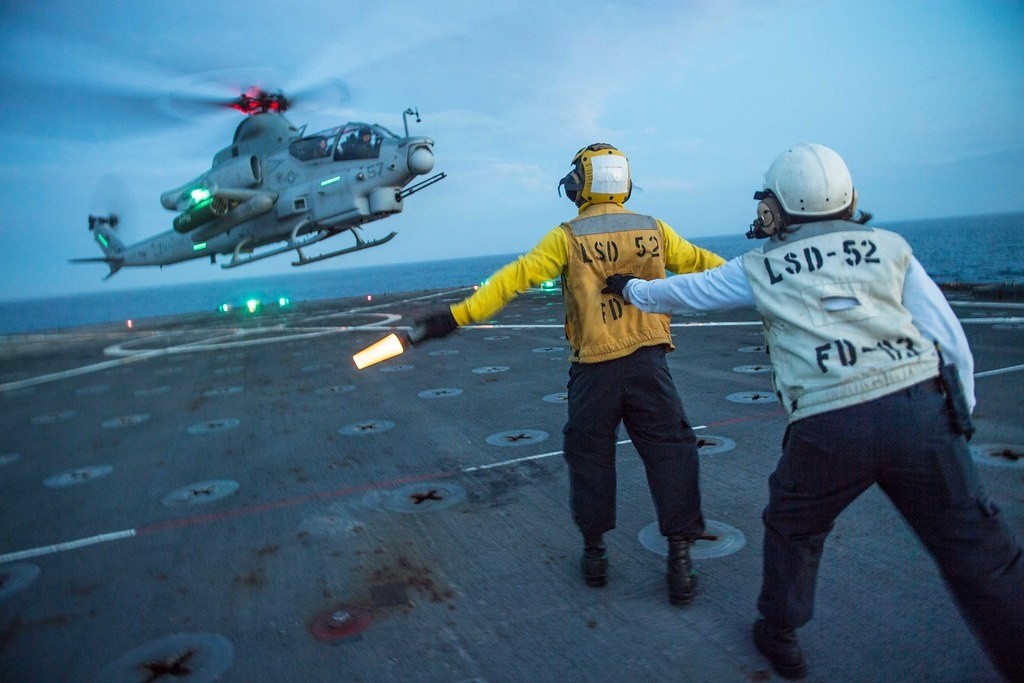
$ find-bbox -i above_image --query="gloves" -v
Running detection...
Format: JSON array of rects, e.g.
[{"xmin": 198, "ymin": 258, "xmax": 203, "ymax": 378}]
[
  {"xmin": 408, "ymin": 306, "xmax": 457, "ymax": 344},
  {"xmin": 601, "ymin": 273, "xmax": 634, "ymax": 305}
]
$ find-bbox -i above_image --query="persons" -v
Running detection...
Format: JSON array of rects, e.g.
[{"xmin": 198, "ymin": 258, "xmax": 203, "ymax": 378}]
[
  {"xmin": 604, "ymin": 146, "xmax": 1024, "ymax": 683},
  {"xmin": 413, "ymin": 143, "xmax": 731, "ymax": 606},
  {"xmin": 350, "ymin": 129, "xmax": 374, "ymax": 157},
  {"xmin": 310, "ymin": 136, "xmax": 328, "ymax": 158}
]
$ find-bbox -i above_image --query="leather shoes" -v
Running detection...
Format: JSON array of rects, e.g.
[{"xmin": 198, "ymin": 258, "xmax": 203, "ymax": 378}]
[
  {"xmin": 583, "ymin": 543, "xmax": 609, "ymax": 587},
  {"xmin": 668, "ymin": 567, "xmax": 699, "ymax": 604}
]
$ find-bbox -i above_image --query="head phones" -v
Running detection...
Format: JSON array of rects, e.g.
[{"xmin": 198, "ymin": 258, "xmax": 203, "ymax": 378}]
[
  {"xmin": 563, "ymin": 169, "xmax": 583, "ymax": 204},
  {"xmin": 756, "ymin": 186, "xmax": 857, "ymax": 236}
]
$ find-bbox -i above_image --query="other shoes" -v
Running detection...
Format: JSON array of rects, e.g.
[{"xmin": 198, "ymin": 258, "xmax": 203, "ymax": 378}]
[{"xmin": 752, "ymin": 619, "xmax": 804, "ymax": 677}]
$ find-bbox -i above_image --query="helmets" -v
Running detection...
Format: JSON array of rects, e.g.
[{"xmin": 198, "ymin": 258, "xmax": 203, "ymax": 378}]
[
  {"xmin": 763, "ymin": 143, "xmax": 853, "ymax": 216},
  {"xmin": 571, "ymin": 142, "xmax": 631, "ymax": 205}
]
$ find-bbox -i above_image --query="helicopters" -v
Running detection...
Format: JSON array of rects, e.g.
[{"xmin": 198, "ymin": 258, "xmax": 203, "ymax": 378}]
[{"xmin": 71, "ymin": 50, "xmax": 449, "ymax": 282}]
